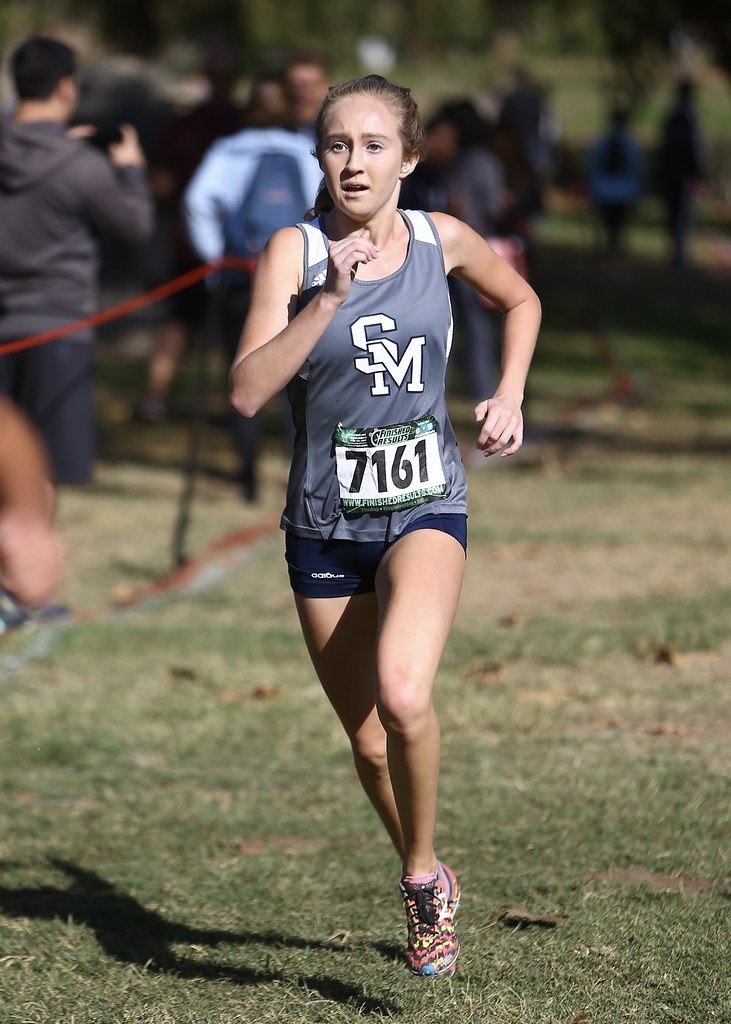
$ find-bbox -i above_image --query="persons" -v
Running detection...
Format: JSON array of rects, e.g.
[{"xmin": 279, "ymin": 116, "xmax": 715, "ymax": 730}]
[
  {"xmin": 230, "ymin": 72, "xmax": 542, "ymax": 976},
  {"xmin": 0, "ymin": 23, "xmax": 700, "ymax": 635}
]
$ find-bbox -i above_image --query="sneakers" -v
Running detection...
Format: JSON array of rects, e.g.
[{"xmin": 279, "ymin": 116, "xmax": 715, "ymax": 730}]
[{"xmin": 400, "ymin": 860, "xmax": 461, "ymax": 977}]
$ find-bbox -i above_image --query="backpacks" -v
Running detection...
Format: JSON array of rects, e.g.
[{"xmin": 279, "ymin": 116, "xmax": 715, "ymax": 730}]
[
  {"xmin": 219, "ymin": 150, "xmax": 310, "ymax": 286},
  {"xmin": 598, "ymin": 135, "xmax": 628, "ymax": 180}
]
